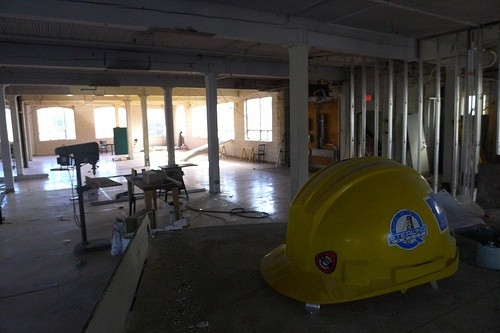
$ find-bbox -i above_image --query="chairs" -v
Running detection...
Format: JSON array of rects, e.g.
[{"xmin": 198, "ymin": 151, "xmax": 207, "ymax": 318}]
[
  {"xmin": 253, "ymin": 144, "xmax": 266, "ymax": 162},
  {"xmin": 452, "ymin": 163, "xmax": 500, "ymax": 248}
]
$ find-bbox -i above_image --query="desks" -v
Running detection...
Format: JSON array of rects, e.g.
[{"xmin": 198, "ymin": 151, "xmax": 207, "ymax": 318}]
[
  {"xmin": 124, "ymin": 173, "xmax": 182, "ymax": 226},
  {"xmin": 125, "ymin": 222, "xmax": 500, "ymax": 333}
]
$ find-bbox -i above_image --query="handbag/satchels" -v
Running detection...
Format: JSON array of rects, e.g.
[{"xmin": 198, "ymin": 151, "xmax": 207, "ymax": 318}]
[{"xmin": 110, "ymin": 218, "xmax": 127, "ymax": 258}]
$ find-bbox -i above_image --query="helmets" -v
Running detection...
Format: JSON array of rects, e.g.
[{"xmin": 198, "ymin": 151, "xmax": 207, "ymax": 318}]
[{"xmin": 260, "ymin": 156, "xmax": 461, "ymax": 304}]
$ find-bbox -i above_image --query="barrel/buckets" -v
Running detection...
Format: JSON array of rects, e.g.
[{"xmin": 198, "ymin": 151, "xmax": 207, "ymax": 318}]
[{"xmin": 169, "ymin": 200, "xmax": 184, "ymax": 224}]
